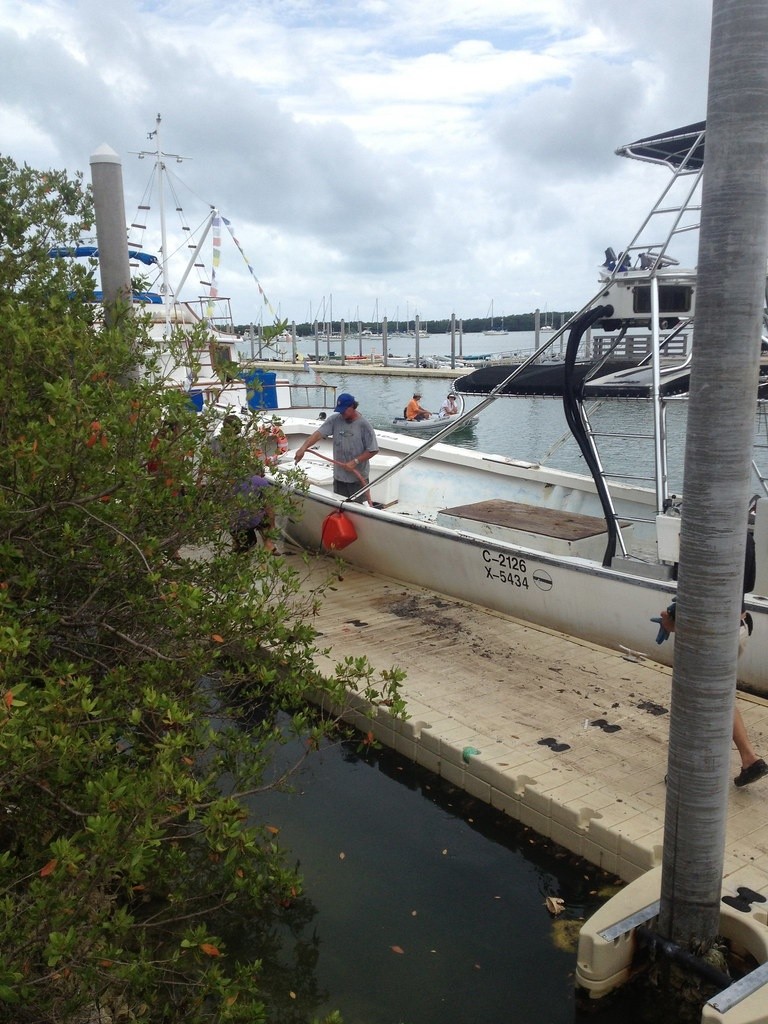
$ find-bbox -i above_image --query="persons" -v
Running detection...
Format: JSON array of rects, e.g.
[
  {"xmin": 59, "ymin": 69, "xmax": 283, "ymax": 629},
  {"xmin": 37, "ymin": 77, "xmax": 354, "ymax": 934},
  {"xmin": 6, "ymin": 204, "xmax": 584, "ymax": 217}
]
[
  {"xmin": 649, "ymin": 529, "xmax": 768, "ymax": 788},
  {"xmin": 405, "ymin": 391, "xmax": 433, "ymax": 421},
  {"xmin": 208, "ymin": 416, "xmax": 281, "ymax": 557},
  {"xmin": 439, "ymin": 393, "xmax": 459, "ymax": 418},
  {"xmin": 148, "ymin": 417, "xmax": 186, "ymax": 565},
  {"xmin": 294, "ymin": 393, "xmax": 379, "ymax": 506}
]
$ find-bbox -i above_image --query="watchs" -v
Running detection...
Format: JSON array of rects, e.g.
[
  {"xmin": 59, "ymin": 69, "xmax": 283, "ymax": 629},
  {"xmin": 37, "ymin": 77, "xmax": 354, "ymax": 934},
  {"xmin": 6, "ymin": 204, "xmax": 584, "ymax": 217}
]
[{"xmin": 354, "ymin": 458, "xmax": 359, "ymax": 464}]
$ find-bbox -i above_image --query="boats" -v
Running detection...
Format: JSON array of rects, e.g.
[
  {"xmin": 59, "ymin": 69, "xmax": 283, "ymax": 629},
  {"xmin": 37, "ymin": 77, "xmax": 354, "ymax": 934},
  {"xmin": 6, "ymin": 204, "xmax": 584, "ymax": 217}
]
[
  {"xmin": 453, "ymin": 247, "xmax": 768, "ymax": 402},
  {"xmin": 391, "ymin": 413, "xmax": 480, "ymax": 430},
  {"xmin": 307, "ymin": 351, "xmax": 491, "ymax": 369}
]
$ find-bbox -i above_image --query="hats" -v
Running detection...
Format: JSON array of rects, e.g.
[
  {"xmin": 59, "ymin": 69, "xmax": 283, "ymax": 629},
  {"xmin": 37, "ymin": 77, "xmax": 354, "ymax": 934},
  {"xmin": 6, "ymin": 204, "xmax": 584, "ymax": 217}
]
[
  {"xmin": 413, "ymin": 393, "xmax": 422, "ymax": 398},
  {"xmin": 333, "ymin": 393, "xmax": 355, "ymax": 412},
  {"xmin": 447, "ymin": 391, "xmax": 457, "ymax": 400}
]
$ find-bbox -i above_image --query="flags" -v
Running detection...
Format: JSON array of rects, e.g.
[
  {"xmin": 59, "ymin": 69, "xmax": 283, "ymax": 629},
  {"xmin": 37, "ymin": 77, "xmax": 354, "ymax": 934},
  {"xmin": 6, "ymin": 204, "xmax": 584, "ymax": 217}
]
[
  {"xmin": 223, "ymin": 215, "xmax": 322, "ymax": 386},
  {"xmin": 207, "ymin": 218, "xmax": 223, "ymax": 320}
]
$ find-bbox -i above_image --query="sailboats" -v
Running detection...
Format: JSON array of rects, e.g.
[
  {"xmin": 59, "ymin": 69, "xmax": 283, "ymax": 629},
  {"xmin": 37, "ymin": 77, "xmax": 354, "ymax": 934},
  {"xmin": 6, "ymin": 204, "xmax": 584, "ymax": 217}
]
[
  {"xmin": 302, "ymin": 294, "xmax": 430, "ymax": 341},
  {"xmin": 269, "ymin": 301, "xmax": 302, "ymax": 341},
  {"xmin": 240, "ymin": 306, "xmax": 265, "ymax": 340},
  {"xmin": 481, "ymin": 300, "xmax": 509, "ymax": 335},
  {"xmin": 45, "ymin": 113, "xmax": 768, "ymax": 699},
  {"xmin": 540, "ymin": 300, "xmax": 555, "ymax": 332},
  {"xmin": 447, "ymin": 315, "xmax": 464, "ymax": 335}
]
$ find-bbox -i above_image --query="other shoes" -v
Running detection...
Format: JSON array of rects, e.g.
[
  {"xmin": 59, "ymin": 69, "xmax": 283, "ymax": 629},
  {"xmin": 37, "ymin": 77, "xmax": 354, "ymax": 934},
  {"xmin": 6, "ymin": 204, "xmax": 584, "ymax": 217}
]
[{"xmin": 263, "ymin": 548, "xmax": 281, "ymax": 556}]
